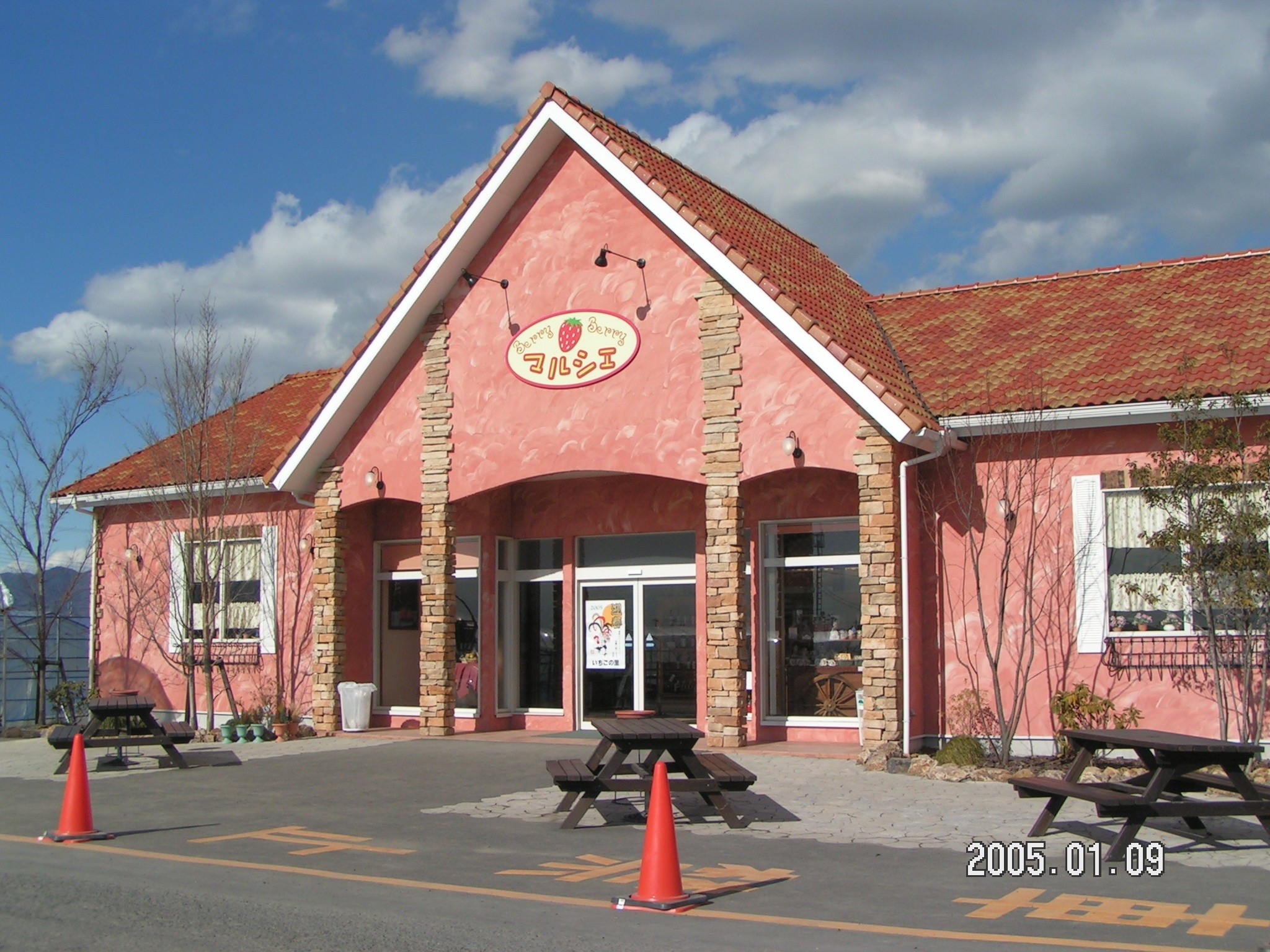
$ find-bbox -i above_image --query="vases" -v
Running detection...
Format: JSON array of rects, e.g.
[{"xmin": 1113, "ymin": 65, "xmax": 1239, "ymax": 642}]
[
  {"xmin": 221, "ymin": 725, "xmax": 232, "ymax": 743},
  {"xmin": 1163, "ymin": 624, "xmax": 1176, "ymax": 631},
  {"xmin": 1136, "ymin": 622, "xmax": 1148, "ymax": 631},
  {"xmin": 1113, "ymin": 625, "xmax": 1122, "ymax": 631}
]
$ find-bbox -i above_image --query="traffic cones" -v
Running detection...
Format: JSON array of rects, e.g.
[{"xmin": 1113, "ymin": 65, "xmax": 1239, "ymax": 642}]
[
  {"xmin": 38, "ymin": 732, "xmax": 118, "ymax": 845},
  {"xmin": 608, "ymin": 761, "xmax": 709, "ymax": 915}
]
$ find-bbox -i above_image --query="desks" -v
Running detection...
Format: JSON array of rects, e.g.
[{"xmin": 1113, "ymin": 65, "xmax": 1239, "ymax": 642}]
[
  {"xmin": 1029, "ymin": 729, "xmax": 1270, "ymax": 861},
  {"xmin": 557, "ymin": 718, "xmax": 746, "ymax": 829},
  {"xmin": 54, "ymin": 696, "xmax": 189, "ymax": 774}
]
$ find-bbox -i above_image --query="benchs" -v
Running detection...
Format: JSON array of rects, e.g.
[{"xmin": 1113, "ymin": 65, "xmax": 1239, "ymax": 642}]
[
  {"xmin": 47, "ymin": 725, "xmax": 82, "ymax": 743},
  {"xmin": 1009, "ymin": 776, "xmax": 1149, "ymax": 807},
  {"xmin": 546, "ymin": 759, "xmax": 595, "ymax": 782},
  {"xmin": 162, "ymin": 721, "xmax": 195, "ymax": 738},
  {"xmin": 1172, "ymin": 772, "xmax": 1270, "ymax": 800},
  {"xmin": 696, "ymin": 753, "xmax": 756, "ymax": 792}
]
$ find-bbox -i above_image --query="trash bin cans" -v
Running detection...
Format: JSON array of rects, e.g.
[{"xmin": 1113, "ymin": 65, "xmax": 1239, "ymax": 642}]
[{"xmin": 337, "ymin": 682, "xmax": 376, "ymax": 732}]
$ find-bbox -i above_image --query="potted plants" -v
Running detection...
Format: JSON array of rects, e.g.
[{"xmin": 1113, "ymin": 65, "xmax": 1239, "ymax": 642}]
[
  {"xmin": 235, "ymin": 709, "xmax": 249, "ymax": 743},
  {"xmin": 272, "ymin": 706, "xmax": 288, "ymax": 742},
  {"xmin": 288, "ymin": 713, "xmax": 301, "ymax": 740},
  {"xmin": 251, "ymin": 706, "xmax": 265, "ymax": 742}
]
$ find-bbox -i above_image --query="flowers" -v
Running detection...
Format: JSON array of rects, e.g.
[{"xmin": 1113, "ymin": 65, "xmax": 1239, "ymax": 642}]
[
  {"xmin": 1110, "ymin": 615, "xmax": 1127, "ymax": 628},
  {"xmin": 1160, "ymin": 613, "xmax": 1183, "ymax": 630},
  {"xmin": 1132, "ymin": 613, "xmax": 1152, "ymax": 625}
]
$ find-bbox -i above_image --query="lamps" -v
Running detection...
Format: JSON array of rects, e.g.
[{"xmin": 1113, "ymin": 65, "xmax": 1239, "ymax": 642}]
[
  {"xmin": 595, "ymin": 242, "xmax": 645, "ymax": 269},
  {"xmin": 125, "ymin": 543, "xmax": 143, "ymax": 563},
  {"xmin": 461, "ymin": 267, "xmax": 508, "ymax": 289},
  {"xmin": 784, "ymin": 430, "xmax": 803, "ymax": 459},
  {"xmin": 365, "ymin": 466, "xmax": 384, "ymax": 489},
  {"xmin": 299, "ymin": 533, "xmax": 314, "ymax": 555},
  {"xmin": 1000, "ymin": 493, "xmax": 1014, "ymax": 522}
]
